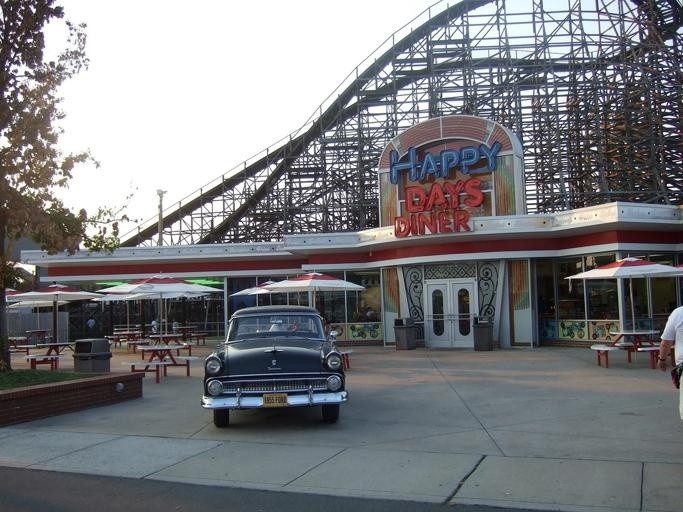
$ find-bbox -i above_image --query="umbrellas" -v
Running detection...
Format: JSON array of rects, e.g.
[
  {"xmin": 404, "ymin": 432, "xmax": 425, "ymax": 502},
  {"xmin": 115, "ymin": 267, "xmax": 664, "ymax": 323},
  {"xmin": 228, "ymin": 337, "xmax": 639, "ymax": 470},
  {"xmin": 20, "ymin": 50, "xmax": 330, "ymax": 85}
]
[
  {"xmin": 95, "ymin": 271, "xmax": 224, "ymax": 383},
  {"xmin": 6, "ymin": 281, "xmax": 106, "ymax": 344},
  {"xmin": 91, "ymin": 293, "xmax": 213, "ymax": 335},
  {"xmin": 229, "ymin": 278, "xmax": 279, "ymax": 305},
  {"xmin": 257, "ymin": 268, "xmax": 366, "ymax": 307},
  {"xmin": 5, "ymin": 288, "xmax": 21, "ymax": 338},
  {"xmin": 563, "ymin": 253, "xmax": 683, "ymax": 362},
  {"xmin": 91, "ymin": 294, "xmax": 140, "ymax": 332},
  {"xmin": 6, "ymin": 300, "xmax": 71, "ymax": 344}
]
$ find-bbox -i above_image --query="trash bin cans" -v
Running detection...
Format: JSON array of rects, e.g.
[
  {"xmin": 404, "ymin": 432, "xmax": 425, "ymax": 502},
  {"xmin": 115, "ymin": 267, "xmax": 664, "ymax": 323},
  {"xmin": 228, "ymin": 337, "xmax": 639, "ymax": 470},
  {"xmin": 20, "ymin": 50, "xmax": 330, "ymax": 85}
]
[
  {"xmin": 72, "ymin": 339, "xmax": 112, "ymax": 372},
  {"xmin": 393, "ymin": 318, "xmax": 419, "ymax": 350},
  {"xmin": 472, "ymin": 316, "xmax": 495, "ymax": 351}
]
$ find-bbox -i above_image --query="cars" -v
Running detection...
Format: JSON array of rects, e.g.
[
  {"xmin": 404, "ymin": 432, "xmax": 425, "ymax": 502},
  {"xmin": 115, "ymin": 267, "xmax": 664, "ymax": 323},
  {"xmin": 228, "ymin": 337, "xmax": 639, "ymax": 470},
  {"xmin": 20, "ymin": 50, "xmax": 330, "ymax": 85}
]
[
  {"xmin": 199, "ymin": 305, "xmax": 349, "ymax": 429},
  {"xmin": 573, "ymin": 278, "xmax": 617, "ymax": 303}
]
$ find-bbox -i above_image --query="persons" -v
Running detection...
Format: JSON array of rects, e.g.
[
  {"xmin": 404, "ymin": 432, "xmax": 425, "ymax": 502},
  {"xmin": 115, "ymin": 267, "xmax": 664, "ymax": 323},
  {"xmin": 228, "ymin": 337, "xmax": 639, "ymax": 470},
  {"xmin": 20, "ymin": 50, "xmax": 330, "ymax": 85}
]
[
  {"xmin": 150, "ymin": 318, "xmax": 157, "ymax": 335},
  {"xmin": 367, "ymin": 307, "xmax": 374, "ymax": 316},
  {"xmin": 172, "ymin": 318, "xmax": 179, "ymax": 334},
  {"xmin": 315, "ymin": 299, "xmax": 355, "ymax": 323},
  {"xmin": 657, "ymin": 306, "xmax": 682, "ymax": 419},
  {"xmin": 86, "ymin": 315, "xmax": 95, "ymax": 337}
]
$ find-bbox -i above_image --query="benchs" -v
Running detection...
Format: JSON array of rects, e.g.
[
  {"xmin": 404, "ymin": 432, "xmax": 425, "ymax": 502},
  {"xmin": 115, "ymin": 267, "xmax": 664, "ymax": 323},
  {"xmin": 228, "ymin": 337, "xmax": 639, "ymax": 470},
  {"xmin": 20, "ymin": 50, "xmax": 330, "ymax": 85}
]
[
  {"xmin": 339, "ymin": 348, "xmax": 353, "ymax": 370},
  {"xmin": 4, "ymin": 320, "xmax": 207, "ymax": 384},
  {"xmin": 585, "ymin": 323, "xmax": 675, "ymax": 369}
]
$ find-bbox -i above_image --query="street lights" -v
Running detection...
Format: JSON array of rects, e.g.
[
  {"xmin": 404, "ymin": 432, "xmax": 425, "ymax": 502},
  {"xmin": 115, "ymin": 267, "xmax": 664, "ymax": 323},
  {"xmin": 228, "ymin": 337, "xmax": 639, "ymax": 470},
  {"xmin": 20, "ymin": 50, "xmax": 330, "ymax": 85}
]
[{"xmin": 154, "ymin": 188, "xmax": 168, "ymax": 246}]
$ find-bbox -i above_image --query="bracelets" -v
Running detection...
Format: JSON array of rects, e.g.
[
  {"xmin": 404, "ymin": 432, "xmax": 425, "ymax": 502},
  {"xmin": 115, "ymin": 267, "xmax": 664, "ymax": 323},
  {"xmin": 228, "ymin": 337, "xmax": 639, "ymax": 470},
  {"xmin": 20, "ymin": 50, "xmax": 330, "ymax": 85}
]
[{"xmin": 658, "ymin": 356, "xmax": 666, "ymax": 361}]
